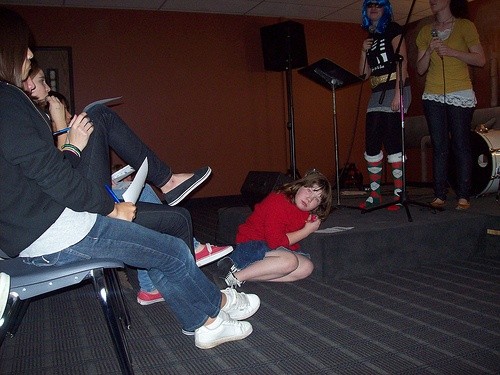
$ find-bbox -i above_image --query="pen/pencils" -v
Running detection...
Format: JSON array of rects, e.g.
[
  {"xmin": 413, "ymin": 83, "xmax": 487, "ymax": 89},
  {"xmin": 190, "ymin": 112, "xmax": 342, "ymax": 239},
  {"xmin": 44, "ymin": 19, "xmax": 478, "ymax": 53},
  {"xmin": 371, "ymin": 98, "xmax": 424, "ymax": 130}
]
[
  {"xmin": 53, "ymin": 127, "xmax": 70, "ymax": 136},
  {"xmin": 104, "ymin": 185, "xmax": 120, "ymax": 202}
]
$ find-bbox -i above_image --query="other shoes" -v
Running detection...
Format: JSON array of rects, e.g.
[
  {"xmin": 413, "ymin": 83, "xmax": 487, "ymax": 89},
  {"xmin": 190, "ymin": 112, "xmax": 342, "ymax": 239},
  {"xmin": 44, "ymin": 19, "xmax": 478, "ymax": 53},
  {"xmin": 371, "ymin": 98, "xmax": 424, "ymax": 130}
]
[
  {"xmin": 163, "ymin": 166, "xmax": 212, "ymax": 207},
  {"xmin": 214, "ymin": 256, "xmax": 245, "ymax": 288},
  {"xmin": 430, "ymin": 197, "xmax": 448, "ymax": 207},
  {"xmin": 455, "ymin": 198, "xmax": 470, "ymax": 210}
]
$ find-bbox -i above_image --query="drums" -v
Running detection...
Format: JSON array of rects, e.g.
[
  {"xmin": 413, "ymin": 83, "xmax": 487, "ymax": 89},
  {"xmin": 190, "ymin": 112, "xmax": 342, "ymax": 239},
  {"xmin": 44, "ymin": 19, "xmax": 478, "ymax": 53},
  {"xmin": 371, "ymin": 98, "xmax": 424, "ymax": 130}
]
[{"xmin": 463, "ymin": 125, "xmax": 500, "ymax": 199}]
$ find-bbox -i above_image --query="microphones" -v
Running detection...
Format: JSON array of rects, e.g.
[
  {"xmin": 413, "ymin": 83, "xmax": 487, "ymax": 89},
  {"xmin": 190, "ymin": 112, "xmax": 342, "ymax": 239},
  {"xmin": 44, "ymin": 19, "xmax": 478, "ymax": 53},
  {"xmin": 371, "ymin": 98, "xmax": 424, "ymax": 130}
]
[
  {"xmin": 431, "ymin": 31, "xmax": 443, "ymax": 60},
  {"xmin": 367, "ymin": 25, "xmax": 376, "ymax": 56}
]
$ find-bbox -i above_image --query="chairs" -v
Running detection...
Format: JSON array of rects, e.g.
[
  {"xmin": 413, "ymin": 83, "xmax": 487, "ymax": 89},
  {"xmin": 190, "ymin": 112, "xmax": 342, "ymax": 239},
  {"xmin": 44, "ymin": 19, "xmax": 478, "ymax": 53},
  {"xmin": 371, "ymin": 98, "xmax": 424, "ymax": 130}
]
[{"xmin": 0, "ymin": 250, "xmax": 135, "ymax": 375}]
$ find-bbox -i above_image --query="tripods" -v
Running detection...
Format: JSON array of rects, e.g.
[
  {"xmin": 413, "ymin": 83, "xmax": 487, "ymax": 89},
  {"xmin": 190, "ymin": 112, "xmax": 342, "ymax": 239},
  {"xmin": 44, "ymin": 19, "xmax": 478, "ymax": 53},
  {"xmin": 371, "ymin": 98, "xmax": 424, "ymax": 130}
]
[{"xmin": 298, "ymin": 0, "xmax": 445, "ymax": 222}]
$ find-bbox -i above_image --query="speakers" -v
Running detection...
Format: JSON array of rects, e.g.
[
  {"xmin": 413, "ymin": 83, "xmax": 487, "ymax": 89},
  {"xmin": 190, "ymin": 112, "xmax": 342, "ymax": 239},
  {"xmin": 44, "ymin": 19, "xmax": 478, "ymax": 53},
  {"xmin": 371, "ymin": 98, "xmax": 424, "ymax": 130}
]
[
  {"xmin": 258, "ymin": 19, "xmax": 308, "ymax": 70},
  {"xmin": 241, "ymin": 170, "xmax": 293, "ymax": 203}
]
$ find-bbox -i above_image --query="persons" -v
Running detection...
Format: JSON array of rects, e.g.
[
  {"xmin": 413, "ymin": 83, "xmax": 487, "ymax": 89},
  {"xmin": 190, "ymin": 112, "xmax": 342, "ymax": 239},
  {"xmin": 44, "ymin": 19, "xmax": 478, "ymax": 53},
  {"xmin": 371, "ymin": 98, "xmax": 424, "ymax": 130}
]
[
  {"xmin": 416, "ymin": 0, "xmax": 485, "ymax": 210},
  {"xmin": 359, "ymin": 0, "xmax": 412, "ymax": 212},
  {"xmin": 0, "ymin": 10, "xmax": 261, "ymax": 350},
  {"xmin": 213, "ymin": 172, "xmax": 332, "ymax": 291}
]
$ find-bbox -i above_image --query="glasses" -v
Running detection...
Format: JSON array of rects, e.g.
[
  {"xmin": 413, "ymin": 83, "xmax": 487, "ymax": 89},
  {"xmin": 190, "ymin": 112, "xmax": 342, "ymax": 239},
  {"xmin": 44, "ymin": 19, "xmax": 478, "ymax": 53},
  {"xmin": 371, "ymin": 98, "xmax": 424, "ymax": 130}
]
[{"xmin": 367, "ymin": 3, "xmax": 390, "ymax": 8}]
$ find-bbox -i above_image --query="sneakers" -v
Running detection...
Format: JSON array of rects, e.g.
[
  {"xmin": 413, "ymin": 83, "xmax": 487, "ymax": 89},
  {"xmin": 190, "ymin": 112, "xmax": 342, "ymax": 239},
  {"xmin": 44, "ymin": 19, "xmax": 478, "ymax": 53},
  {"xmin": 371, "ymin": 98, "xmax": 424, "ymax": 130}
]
[
  {"xmin": 137, "ymin": 291, "xmax": 165, "ymax": 305},
  {"xmin": 195, "ymin": 310, "xmax": 253, "ymax": 349},
  {"xmin": 220, "ymin": 287, "xmax": 261, "ymax": 320},
  {"xmin": 194, "ymin": 243, "xmax": 234, "ymax": 268}
]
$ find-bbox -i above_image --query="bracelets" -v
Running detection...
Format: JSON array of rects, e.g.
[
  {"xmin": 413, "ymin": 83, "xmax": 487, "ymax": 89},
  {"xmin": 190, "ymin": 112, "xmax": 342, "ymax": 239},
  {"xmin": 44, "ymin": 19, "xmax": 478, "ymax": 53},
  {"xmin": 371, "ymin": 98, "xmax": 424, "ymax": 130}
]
[{"xmin": 61, "ymin": 143, "xmax": 82, "ymax": 157}]
